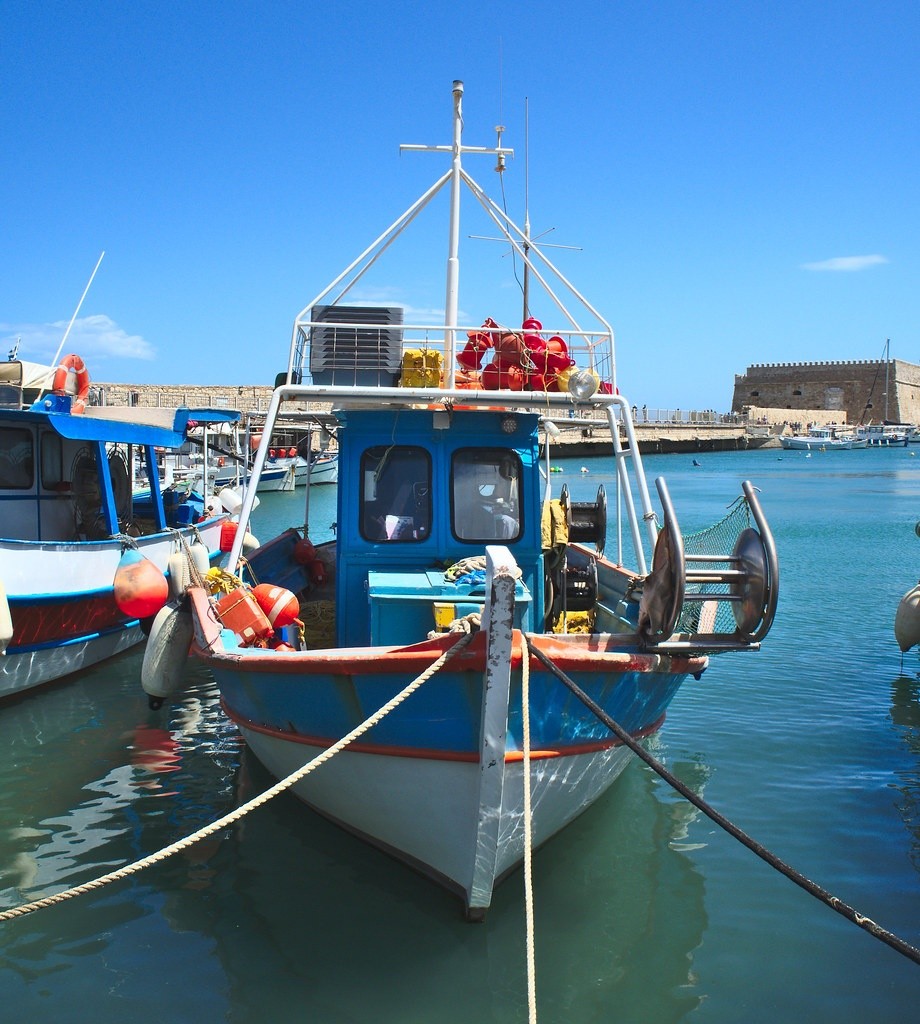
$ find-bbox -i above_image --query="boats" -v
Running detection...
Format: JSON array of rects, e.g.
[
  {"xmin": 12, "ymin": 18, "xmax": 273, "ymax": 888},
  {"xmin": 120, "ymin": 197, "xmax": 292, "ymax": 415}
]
[
  {"xmin": 0, "ymin": 251, "xmax": 340, "ymax": 697},
  {"xmin": 142, "ymin": 79, "xmax": 783, "ymax": 926},
  {"xmin": 779, "ymin": 420, "xmax": 919, "ymax": 450}
]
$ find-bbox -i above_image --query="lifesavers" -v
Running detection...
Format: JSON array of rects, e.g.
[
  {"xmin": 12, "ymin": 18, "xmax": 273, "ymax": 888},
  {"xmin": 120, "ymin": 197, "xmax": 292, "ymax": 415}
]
[{"xmin": 53, "ymin": 355, "xmax": 89, "ymax": 415}]
